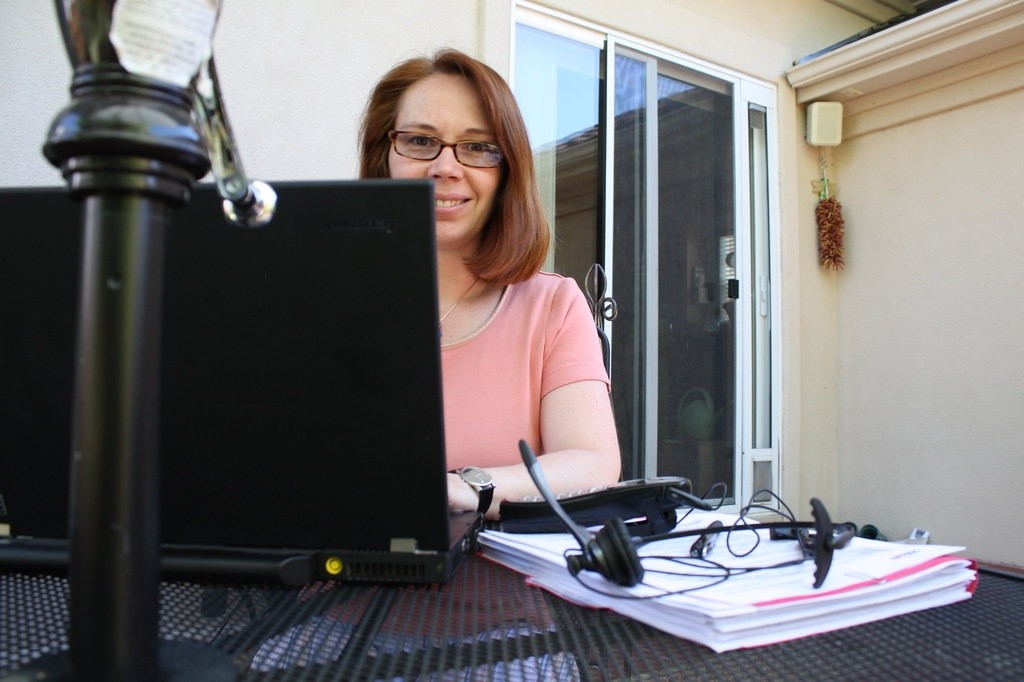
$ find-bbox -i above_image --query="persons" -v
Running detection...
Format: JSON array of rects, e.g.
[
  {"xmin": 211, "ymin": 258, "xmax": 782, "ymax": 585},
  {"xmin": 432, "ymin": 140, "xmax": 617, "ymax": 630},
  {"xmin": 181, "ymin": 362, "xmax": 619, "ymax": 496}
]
[{"xmin": 359, "ymin": 48, "xmax": 620, "ymax": 519}]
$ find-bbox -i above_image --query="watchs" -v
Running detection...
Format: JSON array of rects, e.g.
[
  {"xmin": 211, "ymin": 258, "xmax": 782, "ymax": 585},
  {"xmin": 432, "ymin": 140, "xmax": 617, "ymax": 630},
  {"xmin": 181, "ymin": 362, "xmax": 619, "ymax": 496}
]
[{"xmin": 448, "ymin": 466, "xmax": 496, "ymax": 519}]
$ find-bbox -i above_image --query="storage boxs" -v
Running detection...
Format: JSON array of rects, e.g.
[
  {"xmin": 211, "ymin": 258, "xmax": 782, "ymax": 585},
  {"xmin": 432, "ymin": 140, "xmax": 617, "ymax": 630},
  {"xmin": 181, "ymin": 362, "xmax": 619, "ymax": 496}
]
[{"xmin": 664, "ymin": 437, "xmax": 729, "ymax": 495}]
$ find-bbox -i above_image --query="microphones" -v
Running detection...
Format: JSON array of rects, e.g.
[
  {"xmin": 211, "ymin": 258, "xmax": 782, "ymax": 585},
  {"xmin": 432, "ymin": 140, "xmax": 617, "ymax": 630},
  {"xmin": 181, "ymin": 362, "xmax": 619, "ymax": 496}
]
[{"xmin": 518, "ymin": 438, "xmax": 590, "ymax": 549}]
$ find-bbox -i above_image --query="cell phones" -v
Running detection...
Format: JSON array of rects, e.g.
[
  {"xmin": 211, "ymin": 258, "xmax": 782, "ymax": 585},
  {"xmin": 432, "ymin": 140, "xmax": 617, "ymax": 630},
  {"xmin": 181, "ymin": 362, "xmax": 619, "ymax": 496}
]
[{"xmin": 501, "ymin": 476, "xmax": 693, "ymax": 534}]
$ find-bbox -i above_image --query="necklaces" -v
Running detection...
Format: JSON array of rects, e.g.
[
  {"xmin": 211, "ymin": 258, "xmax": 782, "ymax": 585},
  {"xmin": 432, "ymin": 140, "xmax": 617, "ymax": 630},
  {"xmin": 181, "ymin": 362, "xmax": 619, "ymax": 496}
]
[{"xmin": 440, "ymin": 278, "xmax": 480, "ymax": 323}]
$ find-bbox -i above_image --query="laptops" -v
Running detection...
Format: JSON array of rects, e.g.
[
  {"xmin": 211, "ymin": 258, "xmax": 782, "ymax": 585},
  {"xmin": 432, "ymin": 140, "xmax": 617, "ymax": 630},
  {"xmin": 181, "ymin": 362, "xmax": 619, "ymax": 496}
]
[{"xmin": 0, "ymin": 180, "xmax": 485, "ymax": 584}]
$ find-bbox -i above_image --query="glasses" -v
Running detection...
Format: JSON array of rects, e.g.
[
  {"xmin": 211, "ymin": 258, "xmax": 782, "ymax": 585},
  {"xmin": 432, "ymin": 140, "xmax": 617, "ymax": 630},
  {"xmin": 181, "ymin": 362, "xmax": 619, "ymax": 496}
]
[{"xmin": 388, "ymin": 130, "xmax": 504, "ymax": 168}]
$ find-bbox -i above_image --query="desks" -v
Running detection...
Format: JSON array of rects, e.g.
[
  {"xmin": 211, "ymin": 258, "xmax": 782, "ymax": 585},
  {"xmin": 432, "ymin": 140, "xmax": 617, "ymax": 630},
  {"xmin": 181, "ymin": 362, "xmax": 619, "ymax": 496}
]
[{"xmin": 0, "ymin": 506, "xmax": 1024, "ymax": 682}]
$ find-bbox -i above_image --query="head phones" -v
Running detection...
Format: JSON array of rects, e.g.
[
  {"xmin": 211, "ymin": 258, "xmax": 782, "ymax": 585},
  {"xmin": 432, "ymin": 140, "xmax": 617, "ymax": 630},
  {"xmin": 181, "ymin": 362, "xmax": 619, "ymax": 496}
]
[{"xmin": 565, "ymin": 498, "xmax": 858, "ymax": 588}]
